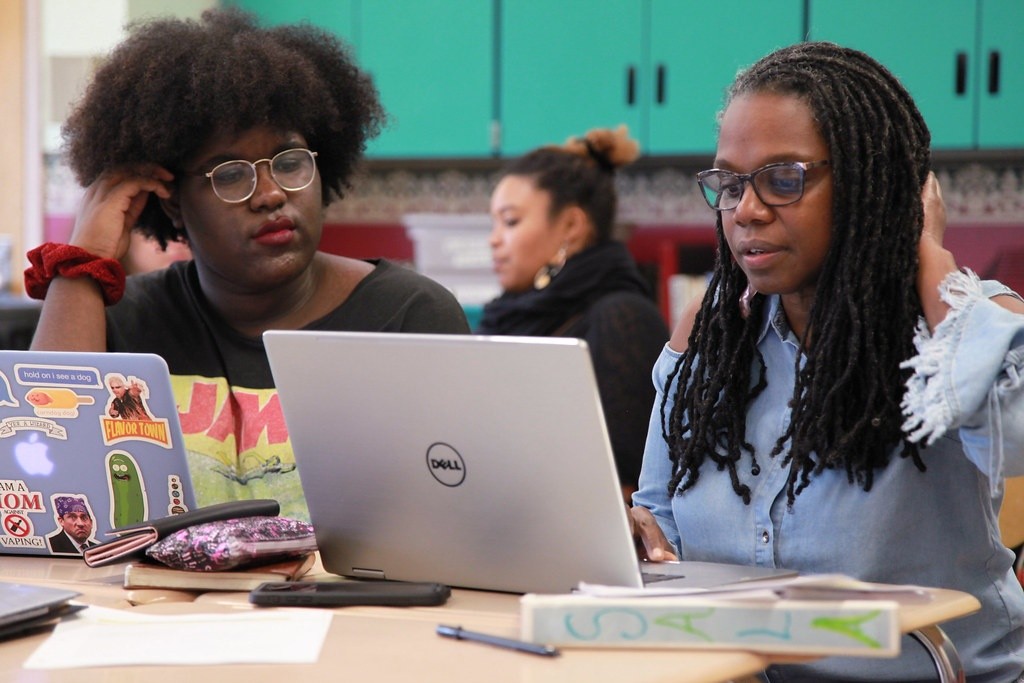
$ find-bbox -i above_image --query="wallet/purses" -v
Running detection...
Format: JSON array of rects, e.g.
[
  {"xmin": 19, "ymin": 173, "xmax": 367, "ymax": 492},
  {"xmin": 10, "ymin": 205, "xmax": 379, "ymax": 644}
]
[
  {"xmin": 144, "ymin": 514, "xmax": 323, "ymax": 569},
  {"xmin": 86, "ymin": 498, "xmax": 280, "ymax": 569}
]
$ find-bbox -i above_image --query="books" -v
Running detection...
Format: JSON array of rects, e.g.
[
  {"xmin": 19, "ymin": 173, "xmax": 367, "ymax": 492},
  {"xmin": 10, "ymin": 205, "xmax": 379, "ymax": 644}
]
[{"xmin": 124, "ymin": 553, "xmax": 317, "ymax": 592}]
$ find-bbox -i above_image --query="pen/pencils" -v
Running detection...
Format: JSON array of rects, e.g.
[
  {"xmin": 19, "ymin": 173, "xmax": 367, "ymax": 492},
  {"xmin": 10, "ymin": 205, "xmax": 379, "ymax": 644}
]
[{"xmin": 437, "ymin": 622, "xmax": 558, "ymax": 657}]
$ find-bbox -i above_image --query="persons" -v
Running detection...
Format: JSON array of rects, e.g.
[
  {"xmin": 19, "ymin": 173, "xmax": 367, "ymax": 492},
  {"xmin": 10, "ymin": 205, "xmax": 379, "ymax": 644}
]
[
  {"xmin": 48, "ymin": 497, "xmax": 100, "ymax": 554},
  {"xmin": 624, "ymin": 43, "xmax": 1024, "ymax": 683},
  {"xmin": 472, "ymin": 127, "xmax": 669, "ymax": 499},
  {"xmin": 26, "ymin": 7, "xmax": 471, "ymax": 522}
]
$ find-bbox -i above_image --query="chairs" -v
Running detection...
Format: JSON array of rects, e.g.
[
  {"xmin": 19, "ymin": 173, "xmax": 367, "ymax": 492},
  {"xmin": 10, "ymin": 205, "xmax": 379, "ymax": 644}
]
[{"xmin": 998, "ymin": 476, "xmax": 1024, "ymax": 578}]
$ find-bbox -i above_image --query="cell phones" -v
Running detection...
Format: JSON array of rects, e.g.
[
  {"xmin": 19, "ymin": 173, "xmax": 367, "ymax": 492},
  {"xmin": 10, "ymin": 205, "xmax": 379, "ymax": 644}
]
[{"xmin": 248, "ymin": 582, "xmax": 451, "ymax": 608}]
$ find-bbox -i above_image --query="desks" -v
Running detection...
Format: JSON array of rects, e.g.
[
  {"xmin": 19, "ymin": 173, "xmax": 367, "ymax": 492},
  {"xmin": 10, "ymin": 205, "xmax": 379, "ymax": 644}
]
[{"xmin": 0, "ymin": 551, "xmax": 983, "ymax": 683}]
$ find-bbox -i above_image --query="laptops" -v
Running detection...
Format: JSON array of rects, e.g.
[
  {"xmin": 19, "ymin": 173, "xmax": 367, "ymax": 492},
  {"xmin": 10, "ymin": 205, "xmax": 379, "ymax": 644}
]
[
  {"xmin": 0, "ymin": 350, "xmax": 197, "ymax": 558},
  {"xmin": 263, "ymin": 327, "xmax": 799, "ymax": 596}
]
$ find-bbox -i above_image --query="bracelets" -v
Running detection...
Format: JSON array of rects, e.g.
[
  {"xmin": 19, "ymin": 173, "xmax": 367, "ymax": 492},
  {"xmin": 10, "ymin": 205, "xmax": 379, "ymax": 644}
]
[{"xmin": 24, "ymin": 244, "xmax": 125, "ymax": 306}]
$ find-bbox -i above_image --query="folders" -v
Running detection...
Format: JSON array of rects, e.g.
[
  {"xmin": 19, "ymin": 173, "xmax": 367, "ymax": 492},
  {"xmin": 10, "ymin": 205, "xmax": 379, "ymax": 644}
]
[{"xmin": 0, "ymin": 579, "xmax": 88, "ymax": 640}]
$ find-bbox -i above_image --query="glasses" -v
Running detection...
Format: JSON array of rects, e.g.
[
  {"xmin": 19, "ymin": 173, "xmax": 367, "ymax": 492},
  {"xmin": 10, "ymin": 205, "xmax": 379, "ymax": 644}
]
[
  {"xmin": 180, "ymin": 148, "xmax": 318, "ymax": 204},
  {"xmin": 695, "ymin": 159, "xmax": 835, "ymax": 211}
]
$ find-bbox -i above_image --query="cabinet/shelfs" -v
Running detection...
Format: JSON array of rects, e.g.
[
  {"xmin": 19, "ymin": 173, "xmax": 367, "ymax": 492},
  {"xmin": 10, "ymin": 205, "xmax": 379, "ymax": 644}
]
[
  {"xmin": 401, "ymin": 212, "xmax": 505, "ymax": 306},
  {"xmin": 807, "ymin": 0, "xmax": 1024, "ymax": 162},
  {"xmin": 499, "ymin": 0, "xmax": 806, "ymax": 161},
  {"xmin": 616, "ymin": 222, "xmax": 721, "ymax": 340},
  {"xmin": 221, "ymin": 0, "xmax": 499, "ymax": 174}
]
